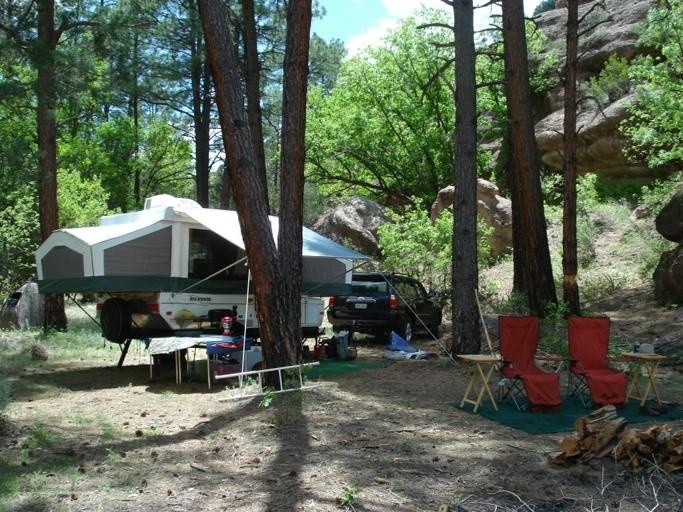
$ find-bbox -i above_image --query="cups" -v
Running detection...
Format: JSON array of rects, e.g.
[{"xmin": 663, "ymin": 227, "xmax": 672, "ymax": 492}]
[{"xmin": 634, "ymin": 343, "xmax": 640, "ymax": 354}]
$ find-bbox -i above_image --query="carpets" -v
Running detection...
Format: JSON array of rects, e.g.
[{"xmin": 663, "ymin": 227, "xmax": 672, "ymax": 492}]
[{"xmin": 449, "ymin": 390, "xmax": 683, "ymax": 435}]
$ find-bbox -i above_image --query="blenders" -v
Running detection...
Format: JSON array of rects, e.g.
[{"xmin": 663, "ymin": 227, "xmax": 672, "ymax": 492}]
[{"xmin": 223, "ymin": 317, "xmax": 232, "ymax": 336}]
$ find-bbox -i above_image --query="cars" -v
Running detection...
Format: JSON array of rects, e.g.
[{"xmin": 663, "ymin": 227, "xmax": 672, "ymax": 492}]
[{"xmin": 327, "ymin": 271, "xmax": 443, "ymax": 343}]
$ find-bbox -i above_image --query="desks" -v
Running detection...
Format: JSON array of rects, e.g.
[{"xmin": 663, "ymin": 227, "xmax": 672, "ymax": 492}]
[
  {"xmin": 147, "ymin": 334, "xmax": 252, "ymax": 391},
  {"xmin": 456, "ymin": 355, "xmax": 501, "ymax": 412},
  {"xmin": 535, "ymin": 356, "xmax": 568, "ymax": 376},
  {"xmin": 621, "ymin": 351, "xmax": 667, "ymax": 407},
  {"xmin": 231, "ymin": 345, "xmax": 264, "ymax": 375}
]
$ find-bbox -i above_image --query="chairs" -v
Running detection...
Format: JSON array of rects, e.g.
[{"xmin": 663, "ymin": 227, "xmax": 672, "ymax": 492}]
[
  {"xmin": 497, "ymin": 314, "xmax": 562, "ymax": 413},
  {"xmin": 300, "ymin": 326, "xmax": 319, "ymax": 345},
  {"xmin": 565, "ymin": 316, "xmax": 626, "ymax": 409}
]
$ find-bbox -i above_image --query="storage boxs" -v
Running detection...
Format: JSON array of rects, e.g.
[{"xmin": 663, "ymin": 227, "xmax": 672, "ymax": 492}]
[
  {"xmin": 188, "ymin": 360, "xmax": 212, "ymax": 382},
  {"xmin": 213, "ymin": 362, "xmax": 242, "ymax": 386}
]
